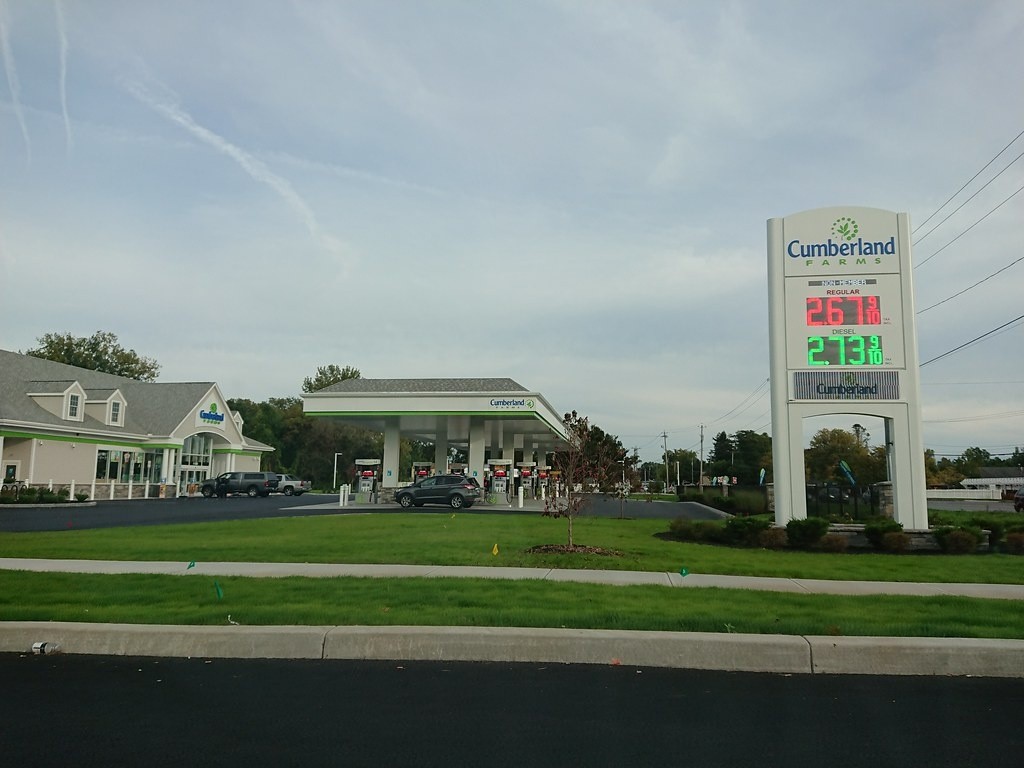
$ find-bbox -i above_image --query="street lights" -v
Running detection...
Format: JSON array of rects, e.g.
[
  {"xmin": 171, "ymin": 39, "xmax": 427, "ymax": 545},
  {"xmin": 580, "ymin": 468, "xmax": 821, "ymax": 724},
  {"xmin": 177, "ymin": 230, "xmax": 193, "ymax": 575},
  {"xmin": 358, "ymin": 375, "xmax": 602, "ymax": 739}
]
[{"xmin": 333, "ymin": 453, "xmax": 343, "ymax": 492}]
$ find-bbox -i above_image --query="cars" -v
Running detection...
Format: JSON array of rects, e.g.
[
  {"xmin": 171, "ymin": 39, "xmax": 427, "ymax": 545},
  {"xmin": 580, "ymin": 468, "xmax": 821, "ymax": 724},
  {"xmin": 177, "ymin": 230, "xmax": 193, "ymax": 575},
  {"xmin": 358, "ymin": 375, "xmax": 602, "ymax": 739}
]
[
  {"xmin": 819, "ymin": 488, "xmax": 850, "ymax": 504},
  {"xmin": 1014, "ymin": 485, "xmax": 1024, "ymax": 512}
]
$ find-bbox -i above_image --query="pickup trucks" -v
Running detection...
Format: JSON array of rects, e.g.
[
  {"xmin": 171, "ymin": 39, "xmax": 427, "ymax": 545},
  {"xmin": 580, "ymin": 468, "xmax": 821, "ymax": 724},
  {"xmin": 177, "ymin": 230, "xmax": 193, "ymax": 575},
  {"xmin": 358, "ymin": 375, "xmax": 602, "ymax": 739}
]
[{"xmin": 276, "ymin": 474, "xmax": 312, "ymax": 497}]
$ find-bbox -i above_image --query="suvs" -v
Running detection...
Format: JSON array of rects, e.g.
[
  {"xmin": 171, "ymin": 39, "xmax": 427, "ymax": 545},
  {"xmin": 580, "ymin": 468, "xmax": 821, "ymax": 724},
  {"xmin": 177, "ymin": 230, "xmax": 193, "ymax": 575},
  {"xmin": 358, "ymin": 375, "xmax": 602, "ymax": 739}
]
[{"xmin": 393, "ymin": 474, "xmax": 481, "ymax": 509}]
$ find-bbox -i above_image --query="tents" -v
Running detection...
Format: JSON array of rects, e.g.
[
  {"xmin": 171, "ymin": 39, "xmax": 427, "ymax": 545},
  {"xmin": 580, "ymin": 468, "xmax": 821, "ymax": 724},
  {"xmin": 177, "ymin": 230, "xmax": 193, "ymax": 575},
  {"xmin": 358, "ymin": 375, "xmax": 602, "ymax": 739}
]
[{"xmin": 960, "ymin": 478, "xmax": 1024, "ymax": 491}]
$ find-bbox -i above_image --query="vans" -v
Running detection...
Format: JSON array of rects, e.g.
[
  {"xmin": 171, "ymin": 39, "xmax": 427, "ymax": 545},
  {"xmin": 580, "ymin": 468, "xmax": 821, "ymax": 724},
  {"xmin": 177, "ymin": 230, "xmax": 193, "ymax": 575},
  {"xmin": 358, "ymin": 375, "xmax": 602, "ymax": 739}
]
[{"xmin": 200, "ymin": 472, "xmax": 279, "ymax": 498}]
